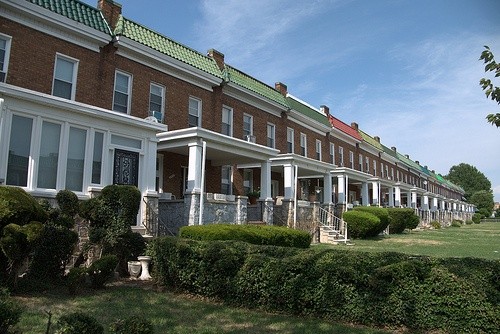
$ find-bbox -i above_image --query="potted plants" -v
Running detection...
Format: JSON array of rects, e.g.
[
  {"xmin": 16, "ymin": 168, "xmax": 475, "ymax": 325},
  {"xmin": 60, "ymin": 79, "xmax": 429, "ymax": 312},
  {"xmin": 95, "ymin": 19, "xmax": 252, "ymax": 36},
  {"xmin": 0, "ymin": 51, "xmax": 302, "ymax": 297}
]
[{"xmin": 247, "ymin": 190, "xmax": 260, "ymax": 205}]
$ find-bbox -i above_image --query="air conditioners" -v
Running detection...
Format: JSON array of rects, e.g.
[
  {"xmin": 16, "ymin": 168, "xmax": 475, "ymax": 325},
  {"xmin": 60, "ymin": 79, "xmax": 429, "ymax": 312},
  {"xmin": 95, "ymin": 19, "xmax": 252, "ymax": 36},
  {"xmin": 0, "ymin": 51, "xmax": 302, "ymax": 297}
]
[{"xmin": 245, "ymin": 136, "xmax": 256, "ymax": 143}]
